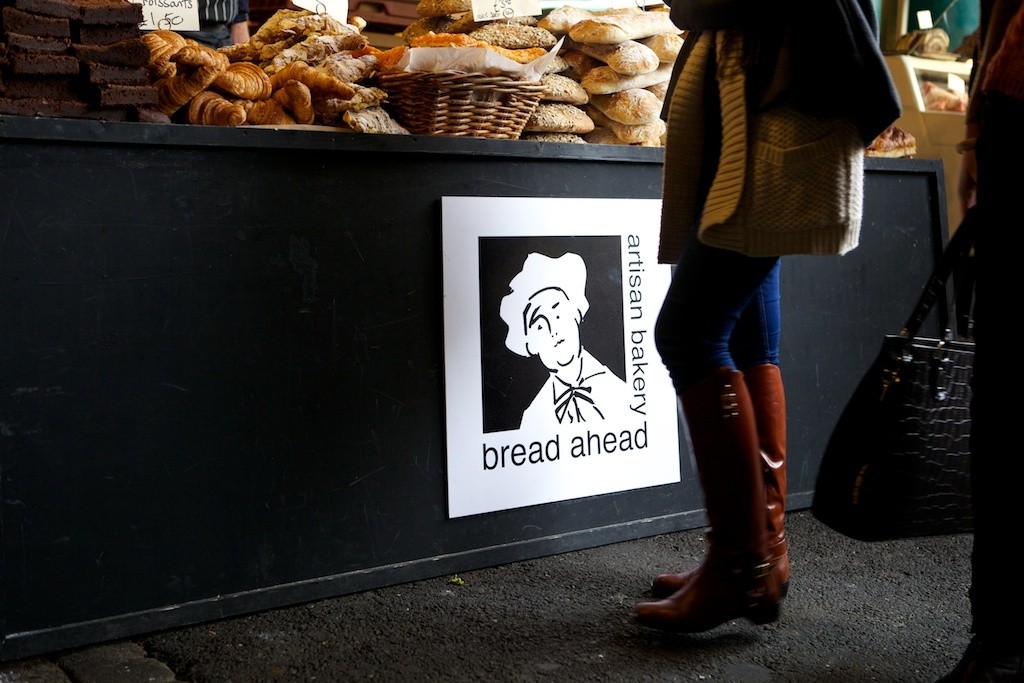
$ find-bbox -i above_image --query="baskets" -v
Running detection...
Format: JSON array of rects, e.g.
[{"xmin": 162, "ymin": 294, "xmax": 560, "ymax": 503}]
[{"xmin": 359, "ymin": 70, "xmax": 547, "ymax": 139}]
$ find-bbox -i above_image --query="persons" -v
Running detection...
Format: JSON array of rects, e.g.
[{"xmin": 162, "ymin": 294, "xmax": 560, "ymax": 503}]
[
  {"xmin": 125, "ymin": 0, "xmax": 250, "ymax": 50},
  {"xmin": 935, "ymin": 0, "xmax": 1024, "ymax": 683},
  {"xmin": 634, "ymin": 1, "xmax": 902, "ymax": 631}
]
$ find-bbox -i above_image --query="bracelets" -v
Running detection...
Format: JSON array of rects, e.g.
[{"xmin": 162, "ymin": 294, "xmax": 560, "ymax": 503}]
[{"xmin": 956, "ymin": 137, "xmax": 977, "ymax": 154}]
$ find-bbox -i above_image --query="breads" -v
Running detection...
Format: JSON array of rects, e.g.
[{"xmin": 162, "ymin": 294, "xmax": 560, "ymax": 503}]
[
  {"xmin": 0, "ymin": 0, "xmax": 412, "ymax": 138},
  {"xmin": 921, "ymin": 78, "xmax": 969, "ymax": 112},
  {"xmin": 396, "ymin": 0, "xmax": 692, "ymax": 150},
  {"xmin": 866, "ymin": 125, "xmax": 918, "ymax": 159},
  {"xmin": 896, "ymin": 29, "xmax": 951, "ymax": 56}
]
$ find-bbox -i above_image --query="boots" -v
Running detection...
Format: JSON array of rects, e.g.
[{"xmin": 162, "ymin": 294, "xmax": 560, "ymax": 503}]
[
  {"xmin": 654, "ymin": 363, "xmax": 788, "ymax": 601},
  {"xmin": 633, "ymin": 367, "xmax": 782, "ymax": 631}
]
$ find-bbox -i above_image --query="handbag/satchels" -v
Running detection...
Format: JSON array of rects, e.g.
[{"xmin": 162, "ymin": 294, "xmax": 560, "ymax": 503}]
[{"xmin": 808, "ymin": 207, "xmax": 977, "ymax": 543}]
